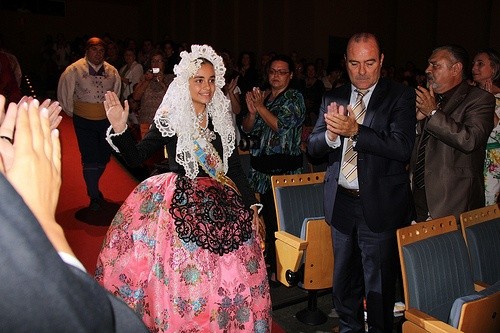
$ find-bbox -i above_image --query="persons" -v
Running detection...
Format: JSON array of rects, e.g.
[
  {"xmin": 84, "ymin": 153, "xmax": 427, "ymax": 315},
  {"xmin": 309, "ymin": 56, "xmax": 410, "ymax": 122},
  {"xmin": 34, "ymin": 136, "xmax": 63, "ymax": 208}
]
[
  {"xmin": 307, "ymin": 32, "xmax": 417, "ymax": 333},
  {"xmin": 239, "ymin": 56, "xmax": 307, "ymax": 283},
  {"xmin": 0, "ymin": 32, "xmax": 500, "ymax": 215},
  {"xmin": 95, "ymin": 44, "xmax": 272, "ymax": 333},
  {"xmin": 0, "ymin": 95, "xmax": 150, "ymax": 333},
  {"xmin": 407, "ymin": 45, "xmax": 496, "ymax": 222}
]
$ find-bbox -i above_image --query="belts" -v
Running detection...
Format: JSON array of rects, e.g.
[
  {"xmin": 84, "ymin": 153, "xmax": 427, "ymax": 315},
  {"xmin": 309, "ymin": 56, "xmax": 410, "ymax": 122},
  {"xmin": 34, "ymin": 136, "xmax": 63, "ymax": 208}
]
[{"xmin": 337, "ymin": 187, "xmax": 360, "ymax": 198}]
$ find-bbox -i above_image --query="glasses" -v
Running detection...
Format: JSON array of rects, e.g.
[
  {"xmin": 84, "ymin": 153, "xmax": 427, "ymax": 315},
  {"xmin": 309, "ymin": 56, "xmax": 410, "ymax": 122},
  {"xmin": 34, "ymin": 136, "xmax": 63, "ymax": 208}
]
[{"xmin": 267, "ymin": 69, "xmax": 289, "ymax": 75}]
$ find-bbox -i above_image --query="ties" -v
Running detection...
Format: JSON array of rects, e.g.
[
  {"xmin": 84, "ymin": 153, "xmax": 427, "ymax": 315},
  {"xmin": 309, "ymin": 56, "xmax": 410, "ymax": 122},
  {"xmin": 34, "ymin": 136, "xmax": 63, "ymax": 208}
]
[
  {"xmin": 415, "ymin": 95, "xmax": 442, "ymax": 189},
  {"xmin": 341, "ymin": 89, "xmax": 370, "ymax": 182}
]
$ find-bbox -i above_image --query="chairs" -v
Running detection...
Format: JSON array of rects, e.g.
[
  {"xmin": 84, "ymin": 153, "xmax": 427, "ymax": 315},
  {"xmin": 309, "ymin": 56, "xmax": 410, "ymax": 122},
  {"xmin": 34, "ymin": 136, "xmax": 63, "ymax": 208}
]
[
  {"xmin": 271, "ymin": 170, "xmax": 335, "ymax": 327},
  {"xmin": 395, "ymin": 205, "xmax": 500, "ymax": 333}
]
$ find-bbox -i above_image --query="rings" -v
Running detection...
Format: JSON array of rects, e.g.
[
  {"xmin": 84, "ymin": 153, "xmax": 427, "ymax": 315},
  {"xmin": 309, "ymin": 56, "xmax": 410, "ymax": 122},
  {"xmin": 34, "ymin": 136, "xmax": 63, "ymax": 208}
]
[{"xmin": 0, "ymin": 135, "xmax": 13, "ymax": 143}]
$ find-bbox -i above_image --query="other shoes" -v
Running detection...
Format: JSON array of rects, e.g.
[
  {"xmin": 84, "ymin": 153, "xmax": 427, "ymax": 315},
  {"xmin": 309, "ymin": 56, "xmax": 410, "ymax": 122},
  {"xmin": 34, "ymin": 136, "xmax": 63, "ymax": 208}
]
[{"xmin": 90, "ymin": 196, "xmax": 106, "ymax": 215}]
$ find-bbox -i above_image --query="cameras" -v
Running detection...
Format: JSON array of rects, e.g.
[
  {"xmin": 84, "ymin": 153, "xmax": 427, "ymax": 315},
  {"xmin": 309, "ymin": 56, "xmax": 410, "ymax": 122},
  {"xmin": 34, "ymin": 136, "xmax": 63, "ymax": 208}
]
[{"xmin": 151, "ymin": 67, "xmax": 160, "ymax": 74}]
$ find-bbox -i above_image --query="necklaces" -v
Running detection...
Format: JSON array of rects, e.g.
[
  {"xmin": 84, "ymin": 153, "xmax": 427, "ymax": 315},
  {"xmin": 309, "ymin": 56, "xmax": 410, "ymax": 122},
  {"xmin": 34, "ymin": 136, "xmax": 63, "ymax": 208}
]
[{"xmin": 191, "ymin": 110, "xmax": 208, "ymax": 123}]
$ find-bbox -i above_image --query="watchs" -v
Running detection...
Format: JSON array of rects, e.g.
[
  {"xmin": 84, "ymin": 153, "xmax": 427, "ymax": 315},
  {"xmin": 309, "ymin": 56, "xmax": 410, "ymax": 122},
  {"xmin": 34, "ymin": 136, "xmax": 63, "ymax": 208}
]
[
  {"xmin": 348, "ymin": 129, "xmax": 359, "ymax": 142},
  {"xmin": 429, "ymin": 108, "xmax": 436, "ymax": 120}
]
